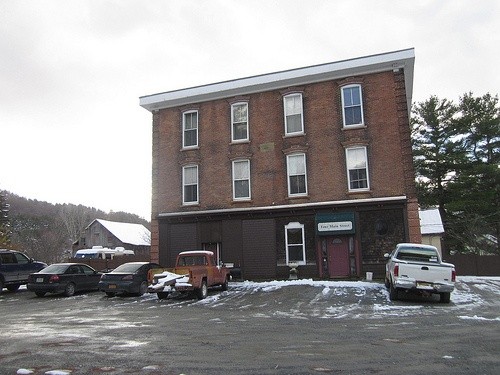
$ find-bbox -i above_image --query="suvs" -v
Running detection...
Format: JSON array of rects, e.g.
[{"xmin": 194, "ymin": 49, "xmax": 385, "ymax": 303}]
[{"xmin": 1, "ymin": 248, "xmax": 48, "ymax": 292}]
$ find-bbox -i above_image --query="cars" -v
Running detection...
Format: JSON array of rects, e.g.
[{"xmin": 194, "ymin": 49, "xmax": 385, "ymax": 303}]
[
  {"xmin": 27, "ymin": 263, "xmax": 103, "ymax": 298},
  {"xmin": 97, "ymin": 262, "xmax": 164, "ymax": 297}
]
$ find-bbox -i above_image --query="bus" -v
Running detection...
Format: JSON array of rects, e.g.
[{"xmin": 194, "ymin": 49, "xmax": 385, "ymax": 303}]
[{"xmin": 74, "ymin": 245, "xmax": 135, "ymax": 264}]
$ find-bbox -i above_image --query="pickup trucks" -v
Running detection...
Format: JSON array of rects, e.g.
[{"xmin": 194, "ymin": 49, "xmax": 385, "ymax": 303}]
[
  {"xmin": 147, "ymin": 249, "xmax": 229, "ymax": 300},
  {"xmin": 383, "ymin": 243, "xmax": 456, "ymax": 302}
]
